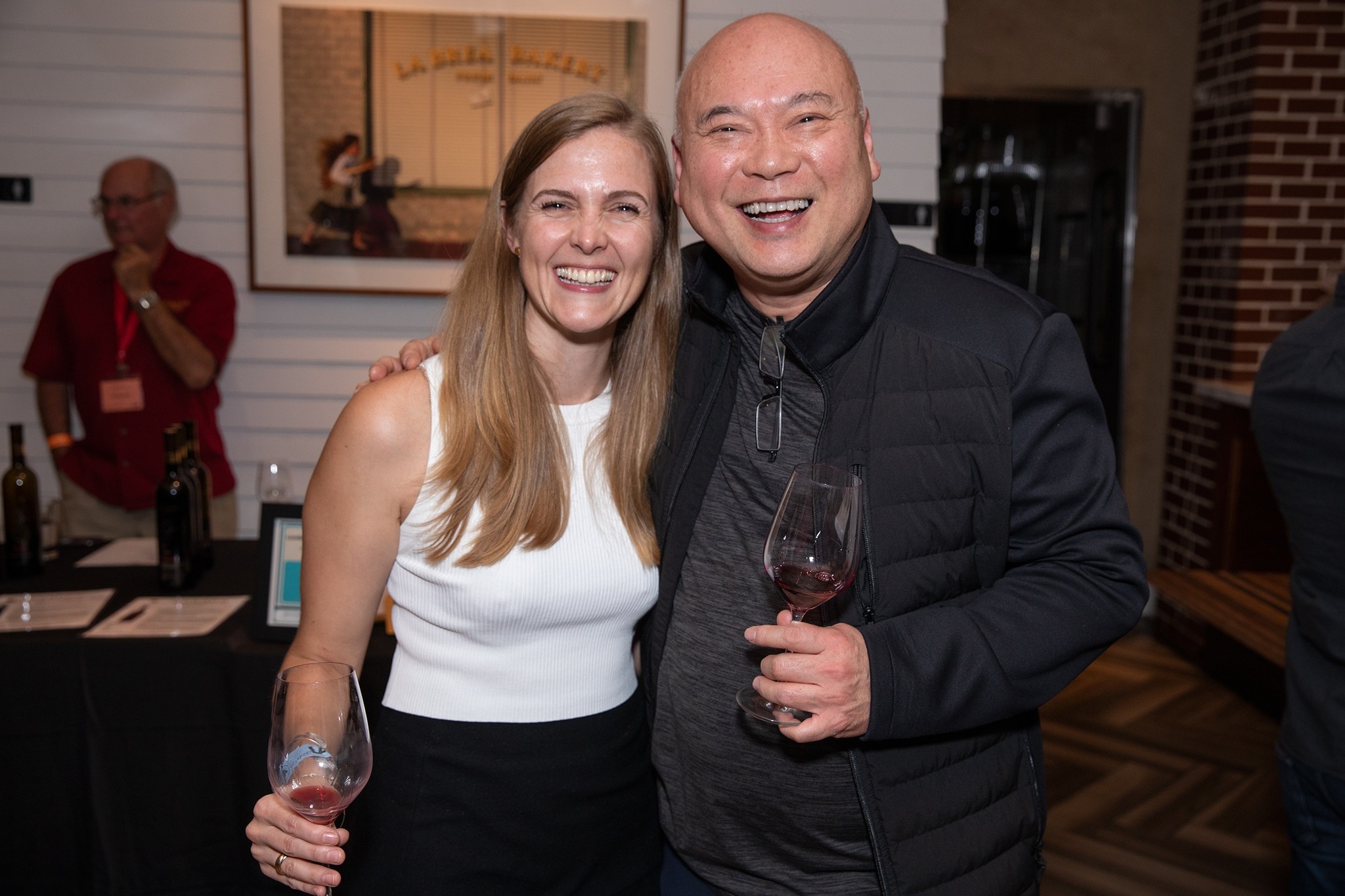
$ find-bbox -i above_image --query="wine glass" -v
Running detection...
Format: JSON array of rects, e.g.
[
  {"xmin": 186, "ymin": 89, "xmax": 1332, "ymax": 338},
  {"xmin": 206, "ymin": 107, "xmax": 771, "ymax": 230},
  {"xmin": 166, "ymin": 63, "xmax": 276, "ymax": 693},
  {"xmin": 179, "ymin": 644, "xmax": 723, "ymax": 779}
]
[
  {"xmin": 735, "ymin": 461, "xmax": 867, "ymax": 723},
  {"xmin": 266, "ymin": 659, "xmax": 373, "ymax": 896},
  {"xmin": 251, "ymin": 459, "xmax": 293, "ymax": 503}
]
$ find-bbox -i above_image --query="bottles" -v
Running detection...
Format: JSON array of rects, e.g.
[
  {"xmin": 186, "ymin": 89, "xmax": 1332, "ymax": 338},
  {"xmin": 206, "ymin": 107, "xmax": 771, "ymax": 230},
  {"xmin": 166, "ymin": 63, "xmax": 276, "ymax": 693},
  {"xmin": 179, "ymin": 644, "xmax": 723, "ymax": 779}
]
[
  {"xmin": 157, "ymin": 417, "xmax": 213, "ymax": 583},
  {"xmin": 3, "ymin": 422, "xmax": 43, "ymax": 577}
]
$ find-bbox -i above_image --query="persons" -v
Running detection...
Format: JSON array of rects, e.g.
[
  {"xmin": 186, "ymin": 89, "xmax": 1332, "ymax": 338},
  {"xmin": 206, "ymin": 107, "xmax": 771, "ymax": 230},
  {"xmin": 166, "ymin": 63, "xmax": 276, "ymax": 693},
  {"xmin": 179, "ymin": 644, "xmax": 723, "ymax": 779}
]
[
  {"xmin": 296, "ymin": 129, "xmax": 377, "ymax": 258},
  {"xmin": 1249, "ymin": 267, "xmax": 1345, "ymax": 895},
  {"xmin": 349, "ymin": 12, "xmax": 1148, "ymax": 896},
  {"xmin": 245, "ymin": 90, "xmax": 679, "ymax": 895},
  {"xmin": 20, "ymin": 156, "xmax": 242, "ymax": 542}
]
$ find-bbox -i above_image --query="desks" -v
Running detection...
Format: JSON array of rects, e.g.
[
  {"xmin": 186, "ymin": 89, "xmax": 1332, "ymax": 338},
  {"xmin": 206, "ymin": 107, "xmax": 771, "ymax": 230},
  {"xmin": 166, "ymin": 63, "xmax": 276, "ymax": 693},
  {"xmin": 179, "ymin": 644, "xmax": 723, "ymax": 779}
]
[{"xmin": 0, "ymin": 539, "xmax": 398, "ymax": 896}]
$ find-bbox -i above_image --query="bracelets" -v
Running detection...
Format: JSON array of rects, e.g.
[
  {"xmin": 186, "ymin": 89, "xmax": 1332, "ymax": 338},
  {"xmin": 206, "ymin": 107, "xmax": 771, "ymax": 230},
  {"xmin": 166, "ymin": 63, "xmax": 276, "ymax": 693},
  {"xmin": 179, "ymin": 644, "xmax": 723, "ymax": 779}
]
[
  {"xmin": 279, "ymin": 744, "xmax": 341, "ymax": 784},
  {"xmin": 46, "ymin": 433, "xmax": 74, "ymax": 449},
  {"xmin": 134, "ymin": 290, "xmax": 157, "ymax": 315}
]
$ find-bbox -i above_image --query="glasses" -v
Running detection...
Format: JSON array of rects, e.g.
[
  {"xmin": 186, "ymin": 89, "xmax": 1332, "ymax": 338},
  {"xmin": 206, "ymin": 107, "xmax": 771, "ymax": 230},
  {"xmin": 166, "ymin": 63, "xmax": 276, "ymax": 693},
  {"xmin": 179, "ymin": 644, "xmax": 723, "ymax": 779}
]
[
  {"xmin": 93, "ymin": 190, "xmax": 169, "ymax": 211},
  {"xmin": 755, "ymin": 315, "xmax": 789, "ymax": 464}
]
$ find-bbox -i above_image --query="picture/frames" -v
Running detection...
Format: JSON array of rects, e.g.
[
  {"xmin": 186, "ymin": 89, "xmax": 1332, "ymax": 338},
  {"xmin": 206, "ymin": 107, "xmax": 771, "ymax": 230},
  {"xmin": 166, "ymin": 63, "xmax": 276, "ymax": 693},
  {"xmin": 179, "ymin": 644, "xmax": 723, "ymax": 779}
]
[
  {"xmin": 237, "ymin": 1, "xmax": 689, "ymax": 296},
  {"xmin": 257, "ymin": 503, "xmax": 303, "ymax": 645}
]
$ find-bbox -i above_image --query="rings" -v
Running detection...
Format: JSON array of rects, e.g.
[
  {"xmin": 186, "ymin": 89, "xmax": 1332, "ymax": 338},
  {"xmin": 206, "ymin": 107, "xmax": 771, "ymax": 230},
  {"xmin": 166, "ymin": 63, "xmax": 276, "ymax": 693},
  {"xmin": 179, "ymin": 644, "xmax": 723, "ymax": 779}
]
[{"xmin": 274, "ymin": 854, "xmax": 288, "ymax": 875}]
